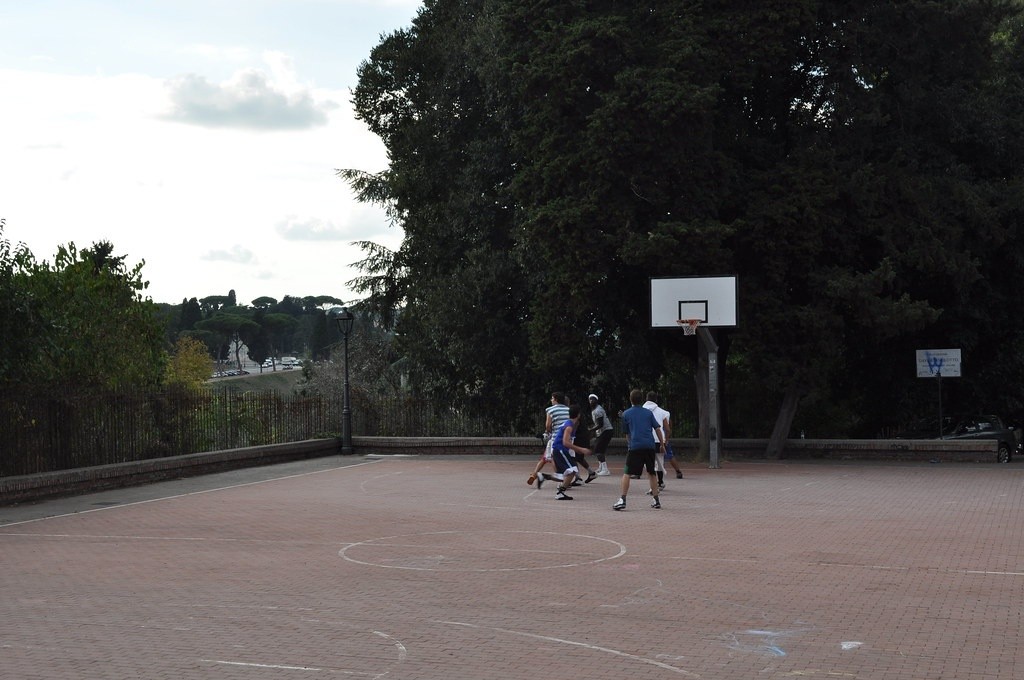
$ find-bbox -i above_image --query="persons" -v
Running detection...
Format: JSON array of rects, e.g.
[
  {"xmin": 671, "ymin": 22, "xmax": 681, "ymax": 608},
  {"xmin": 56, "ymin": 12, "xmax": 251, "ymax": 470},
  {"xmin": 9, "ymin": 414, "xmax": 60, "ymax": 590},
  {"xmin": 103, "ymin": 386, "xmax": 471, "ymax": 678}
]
[
  {"xmin": 536, "ymin": 405, "xmax": 592, "ymax": 500},
  {"xmin": 526, "ymin": 390, "xmax": 599, "ymax": 485},
  {"xmin": 587, "ymin": 394, "xmax": 613, "ymax": 476},
  {"xmin": 613, "ymin": 389, "xmax": 668, "ymax": 510},
  {"xmin": 617, "ymin": 392, "xmax": 683, "ymax": 490}
]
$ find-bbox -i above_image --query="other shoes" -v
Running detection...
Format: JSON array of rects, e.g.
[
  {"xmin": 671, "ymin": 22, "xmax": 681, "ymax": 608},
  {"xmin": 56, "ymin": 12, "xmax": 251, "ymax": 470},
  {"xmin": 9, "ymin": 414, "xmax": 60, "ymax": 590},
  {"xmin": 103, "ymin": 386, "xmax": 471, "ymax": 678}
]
[
  {"xmin": 555, "ymin": 485, "xmax": 573, "ymax": 500},
  {"xmin": 631, "ymin": 475, "xmax": 641, "ymax": 480},
  {"xmin": 537, "ymin": 472, "xmax": 551, "ymax": 489},
  {"xmin": 527, "ymin": 471, "xmax": 537, "ymax": 485},
  {"xmin": 676, "ymin": 472, "xmax": 682, "ymax": 478},
  {"xmin": 585, "ymin": 472, "xmax": 598, "ymax": 483},
  {"xmin": 572, "ymin": 478, "xmax": 582, "ymax": 484}
]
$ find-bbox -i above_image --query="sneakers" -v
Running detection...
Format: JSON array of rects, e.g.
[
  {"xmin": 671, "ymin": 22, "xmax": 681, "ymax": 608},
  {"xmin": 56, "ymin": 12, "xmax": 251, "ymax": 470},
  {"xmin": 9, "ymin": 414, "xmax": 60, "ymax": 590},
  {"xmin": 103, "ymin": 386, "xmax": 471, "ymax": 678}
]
[
  {"xmin": 651, "ymin": 497, "xmax": 660, "ymax": 508},
  {"xmin": 646, "ymin": 487, "xmax": 661, "ymax": 495},
  {"xmin": 613, "ymin": 497, "xmax": 626, "ymax": 509},
  {"xmin": 657, "ymin": 481, "xmax": 666, "ymax": 491}
]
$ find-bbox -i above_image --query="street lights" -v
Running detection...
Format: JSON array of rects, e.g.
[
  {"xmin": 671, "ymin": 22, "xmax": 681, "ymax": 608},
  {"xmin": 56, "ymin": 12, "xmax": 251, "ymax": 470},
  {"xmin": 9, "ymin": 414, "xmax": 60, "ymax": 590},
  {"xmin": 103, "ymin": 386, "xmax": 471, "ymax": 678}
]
[{"xmin": 333, "ymin": 307, "xmax": 354, "ymax": 456}]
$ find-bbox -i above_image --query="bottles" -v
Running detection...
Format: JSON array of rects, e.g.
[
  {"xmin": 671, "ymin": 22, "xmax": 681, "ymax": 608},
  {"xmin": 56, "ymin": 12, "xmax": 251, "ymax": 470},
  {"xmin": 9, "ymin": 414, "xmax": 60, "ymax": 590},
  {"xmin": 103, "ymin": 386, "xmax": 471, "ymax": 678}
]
[{"xmin": 800, "ymin": 429, "xmax": 805, "ymax": 439}]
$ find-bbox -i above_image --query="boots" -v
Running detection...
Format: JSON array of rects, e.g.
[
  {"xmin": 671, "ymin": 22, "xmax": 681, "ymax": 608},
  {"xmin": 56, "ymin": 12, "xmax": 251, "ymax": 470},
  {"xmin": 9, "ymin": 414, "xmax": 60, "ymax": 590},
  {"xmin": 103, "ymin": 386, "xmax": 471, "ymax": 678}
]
[{"xmin": 595, "ymin": 461, "xmax": 609, "ymax": 475}]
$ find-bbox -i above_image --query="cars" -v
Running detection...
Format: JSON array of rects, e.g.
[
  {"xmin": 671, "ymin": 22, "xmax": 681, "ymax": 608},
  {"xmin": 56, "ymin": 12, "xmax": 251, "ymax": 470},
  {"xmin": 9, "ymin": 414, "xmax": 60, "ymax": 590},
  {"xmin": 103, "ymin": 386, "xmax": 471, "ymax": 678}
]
[{"xmin": 894, "ymin": 415, "xmax": 1018, "ymax": 463}]
